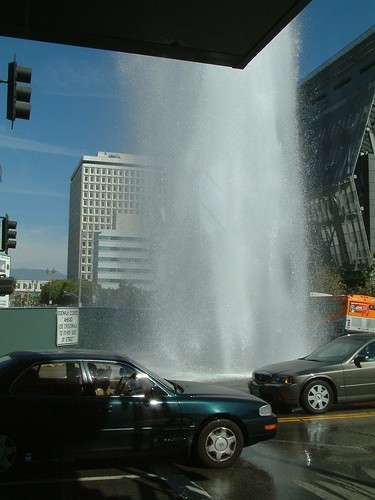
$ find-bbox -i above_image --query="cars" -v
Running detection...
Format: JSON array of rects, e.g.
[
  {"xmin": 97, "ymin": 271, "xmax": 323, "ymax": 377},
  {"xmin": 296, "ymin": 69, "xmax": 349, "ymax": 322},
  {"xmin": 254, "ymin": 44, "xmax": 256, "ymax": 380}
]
[
  {"xmin": 0, "ymin": 349, "xmax": 280, "ymax": 472},
  {"xmin": 248, "ymin": 332, "xmax": 375, "ymax": 414}
]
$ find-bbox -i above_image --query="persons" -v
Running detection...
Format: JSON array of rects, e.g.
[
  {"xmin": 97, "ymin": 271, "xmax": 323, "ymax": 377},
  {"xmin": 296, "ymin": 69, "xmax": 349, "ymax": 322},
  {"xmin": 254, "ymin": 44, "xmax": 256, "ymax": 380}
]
[{"xmin": 89, "ymin": 362, "xmax": 112, "ymax": 396}]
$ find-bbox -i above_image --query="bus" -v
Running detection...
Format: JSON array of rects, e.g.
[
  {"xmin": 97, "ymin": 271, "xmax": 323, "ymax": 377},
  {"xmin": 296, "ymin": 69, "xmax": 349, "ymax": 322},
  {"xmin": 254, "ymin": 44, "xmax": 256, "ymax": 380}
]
[{"xmin": 293, "ymin": 294, "xmax": 374, "ymax": 339}]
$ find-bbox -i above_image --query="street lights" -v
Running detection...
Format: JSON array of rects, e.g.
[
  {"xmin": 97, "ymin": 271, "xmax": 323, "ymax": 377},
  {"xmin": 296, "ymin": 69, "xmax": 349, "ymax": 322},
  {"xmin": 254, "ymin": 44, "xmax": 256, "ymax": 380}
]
[{"xmin": 45, "ymin": 266, "xmax": 56, "ymax": 308}]
